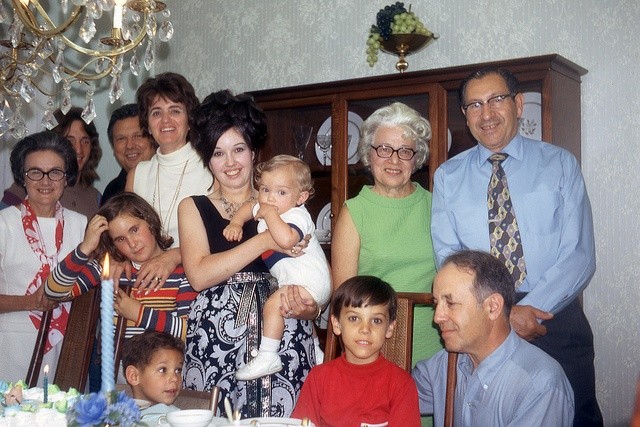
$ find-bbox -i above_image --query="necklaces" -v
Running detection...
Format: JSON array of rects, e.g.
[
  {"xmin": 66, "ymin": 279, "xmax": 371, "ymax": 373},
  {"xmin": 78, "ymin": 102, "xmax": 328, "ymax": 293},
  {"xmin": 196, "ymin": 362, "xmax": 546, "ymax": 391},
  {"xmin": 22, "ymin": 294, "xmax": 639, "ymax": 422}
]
[{"xmin": 218, "ymin": 186, "xmax": 257, "ymax": 220}]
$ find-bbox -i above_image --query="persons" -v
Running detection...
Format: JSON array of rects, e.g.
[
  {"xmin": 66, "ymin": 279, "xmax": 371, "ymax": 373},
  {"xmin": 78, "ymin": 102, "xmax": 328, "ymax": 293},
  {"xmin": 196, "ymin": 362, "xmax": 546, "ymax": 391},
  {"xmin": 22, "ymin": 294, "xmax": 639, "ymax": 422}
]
[
  {"xmin": 126, "ymin": 70, "xmax": 216, "ymax": 250},
  {"xmin": 291, "ymin": 275, "xmax": 422, "ymax": 427},
  {"xmin": 0, "ymin": 129, "xmax": 87, "ymax": 390},
  {"xmin": 99, "ymin": 102, "xmax": 157, "ymax": 205},
  {"xmin": 1, "ymin": 105, "xmax": 103, "ymax": 222},
  {"xmin": 119, "ymin": 331, "xmax": 187, "ymax": 407},
  {"xmin": 429, "ymin": 65, "xmax": 603, "ymax": 427},
  {"xmin": 176, "ymin": 86, "xmax": 339, "ymax": 418},
  {"xmin": 44, "ymin": 190, "xmax": 197, "ymax": 388},
  {"xmin": 223, "ymin": 153, "xmax": 334, "ymax": 382},
  {"xmin": 329, "ymin": 101, "xmax": 446, "ymax": 427},
  {"xmin": 409, "ymin": 250, "xmax": 576, "ymax": 427}
]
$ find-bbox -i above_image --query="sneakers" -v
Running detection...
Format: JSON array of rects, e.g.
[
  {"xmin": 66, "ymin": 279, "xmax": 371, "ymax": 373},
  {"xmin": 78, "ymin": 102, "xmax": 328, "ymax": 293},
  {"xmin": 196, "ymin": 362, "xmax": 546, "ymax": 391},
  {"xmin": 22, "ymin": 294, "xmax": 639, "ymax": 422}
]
[{"xmin": 236, "ymin": 351, "xmax": 282, "ymax": 381}]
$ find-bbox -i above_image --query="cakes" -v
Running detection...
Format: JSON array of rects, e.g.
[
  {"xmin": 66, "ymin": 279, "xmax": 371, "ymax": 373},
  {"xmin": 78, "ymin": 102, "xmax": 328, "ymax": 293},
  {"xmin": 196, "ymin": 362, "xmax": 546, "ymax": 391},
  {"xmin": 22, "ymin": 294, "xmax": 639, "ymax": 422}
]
[{"xmin": 0, "ymin": 364, "xmax": 85, "ymax": 427}]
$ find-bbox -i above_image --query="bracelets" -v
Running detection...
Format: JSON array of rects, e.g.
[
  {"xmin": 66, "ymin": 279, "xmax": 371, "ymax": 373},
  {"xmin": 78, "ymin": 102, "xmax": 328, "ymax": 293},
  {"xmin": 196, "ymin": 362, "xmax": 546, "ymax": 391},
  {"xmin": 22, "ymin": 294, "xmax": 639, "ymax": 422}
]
[{"xmin": 315, "ymin": 305, "xmax": 322, "ymax": 321}]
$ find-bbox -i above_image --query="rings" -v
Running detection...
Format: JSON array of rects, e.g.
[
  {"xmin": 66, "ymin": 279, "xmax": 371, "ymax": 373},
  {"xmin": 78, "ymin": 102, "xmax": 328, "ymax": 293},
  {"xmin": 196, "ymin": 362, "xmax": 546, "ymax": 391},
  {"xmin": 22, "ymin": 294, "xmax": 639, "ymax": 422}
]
[
  {"xmin": 286, "ymin": 310, "xmax": 296, "ymax": 316},
  {"xmin": 153, "ymin": 277, "xmax": 162, "ymax": 282}
]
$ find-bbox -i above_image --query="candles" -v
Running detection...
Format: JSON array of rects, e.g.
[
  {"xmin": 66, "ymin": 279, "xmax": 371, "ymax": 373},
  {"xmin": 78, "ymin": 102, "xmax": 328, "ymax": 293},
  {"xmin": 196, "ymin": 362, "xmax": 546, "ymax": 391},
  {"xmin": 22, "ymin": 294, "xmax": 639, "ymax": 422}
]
[
  {"xmin": 42, "ymin": 363, "xmax": 50, "ymax": 405},
  {"xmin": 98, "ymin": 276, "xmax": 117, "ymax": 410}
]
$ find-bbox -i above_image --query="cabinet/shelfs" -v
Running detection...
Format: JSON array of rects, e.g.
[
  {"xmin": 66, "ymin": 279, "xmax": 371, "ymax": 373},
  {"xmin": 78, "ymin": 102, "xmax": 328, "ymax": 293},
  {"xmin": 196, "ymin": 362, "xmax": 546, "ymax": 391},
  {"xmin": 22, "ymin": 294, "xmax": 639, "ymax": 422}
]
[{"xmin": 231, "ymin": 52, "xmax": 588, "ymax": 353}]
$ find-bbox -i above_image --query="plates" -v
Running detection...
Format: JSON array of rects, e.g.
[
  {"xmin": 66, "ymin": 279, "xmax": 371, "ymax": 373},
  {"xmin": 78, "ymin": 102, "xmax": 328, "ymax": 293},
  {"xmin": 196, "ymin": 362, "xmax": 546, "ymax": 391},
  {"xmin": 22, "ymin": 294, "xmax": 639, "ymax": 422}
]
[
  {"xmin": 315, "ymin": 110, "xmax": 364, "ymax": 166},
  {"xmin": 517, "ymin": 92, "xmax": 542, "ymax": 140},
  {"xmin": 316, "ymin": 202, "xmax": 331, "ymax": 229}
]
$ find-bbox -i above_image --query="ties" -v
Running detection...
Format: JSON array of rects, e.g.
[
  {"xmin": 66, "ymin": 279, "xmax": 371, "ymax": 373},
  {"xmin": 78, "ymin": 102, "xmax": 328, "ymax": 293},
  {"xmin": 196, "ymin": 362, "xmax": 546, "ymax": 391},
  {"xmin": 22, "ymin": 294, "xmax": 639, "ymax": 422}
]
[{"xmin": 487, "ymin": 153, "xmax": 526, "ymax": 290}]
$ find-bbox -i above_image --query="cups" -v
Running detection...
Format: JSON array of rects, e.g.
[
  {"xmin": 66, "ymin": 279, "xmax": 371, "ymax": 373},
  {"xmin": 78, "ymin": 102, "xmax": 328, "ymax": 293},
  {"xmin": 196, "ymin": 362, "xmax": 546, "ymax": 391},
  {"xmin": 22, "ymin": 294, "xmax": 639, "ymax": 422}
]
[{"xmin": 158, "ymin": 408, "xmax": 213, "ymax": 426}]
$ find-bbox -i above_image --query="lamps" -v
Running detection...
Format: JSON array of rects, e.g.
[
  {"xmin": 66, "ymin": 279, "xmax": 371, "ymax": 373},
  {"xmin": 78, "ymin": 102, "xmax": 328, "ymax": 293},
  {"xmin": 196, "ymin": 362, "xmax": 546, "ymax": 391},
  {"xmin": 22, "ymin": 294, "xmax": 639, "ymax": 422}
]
[{"xmin": 0, "ymin": 0, "xmax": 176, "ymax": 142}]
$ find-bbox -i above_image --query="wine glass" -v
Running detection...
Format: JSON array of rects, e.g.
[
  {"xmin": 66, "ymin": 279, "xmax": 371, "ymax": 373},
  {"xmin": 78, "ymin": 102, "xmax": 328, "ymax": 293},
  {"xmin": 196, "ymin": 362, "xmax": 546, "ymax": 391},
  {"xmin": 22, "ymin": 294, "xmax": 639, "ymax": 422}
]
[
  {"xmin": 290, "ymin": 125, "xmax": 313, "ymax": 160},
  {"xmin": 311, "ymin": 134, "xmax": 331, "ymax": 166}
]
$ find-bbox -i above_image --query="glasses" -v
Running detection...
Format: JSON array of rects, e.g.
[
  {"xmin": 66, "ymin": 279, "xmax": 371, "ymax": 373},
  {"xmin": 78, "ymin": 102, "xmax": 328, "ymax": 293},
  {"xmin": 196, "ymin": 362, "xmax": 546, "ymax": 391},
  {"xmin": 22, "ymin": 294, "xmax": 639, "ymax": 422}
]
[
  {"xmin": 464, "ymin": 96, "xmax": 512, "ymax": 111},
  {"xmin": 23, "ymin": 168, "xmax": 66, "ymax": 181},
  {"xmin": 370, "ymin": 146, "xmax": 417, "ymax": 160}
]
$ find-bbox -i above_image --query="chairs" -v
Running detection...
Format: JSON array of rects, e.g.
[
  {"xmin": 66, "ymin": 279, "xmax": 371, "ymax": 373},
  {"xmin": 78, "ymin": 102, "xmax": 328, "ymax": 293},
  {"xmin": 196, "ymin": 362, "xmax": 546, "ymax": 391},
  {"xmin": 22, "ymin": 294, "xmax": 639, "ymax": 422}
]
[
  {"xmin": 112, "ymin": 381, "xmax": 221, "ymax": 424},
  {"xmin": 316, "ymin": 287, "xmax": 461, "ymax": 426},
  {"xmin": 24, "ymin": 273, "xmax": 135, "ymax": 393}
]
